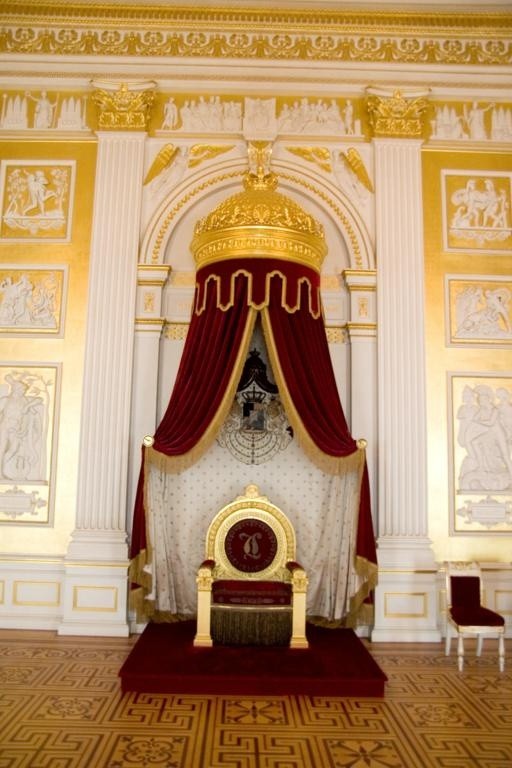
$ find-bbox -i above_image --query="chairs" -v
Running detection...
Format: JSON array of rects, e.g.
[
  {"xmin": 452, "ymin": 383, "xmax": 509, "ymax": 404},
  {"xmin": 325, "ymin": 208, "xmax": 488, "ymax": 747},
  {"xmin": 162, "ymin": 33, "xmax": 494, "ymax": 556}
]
[
  {"xmin": 193, "ymin": 483, "xmax": 312, "ymax": 652},
  {"xmin": 441, "ymin": 565, "xmax": 509, "ymax": 677}
]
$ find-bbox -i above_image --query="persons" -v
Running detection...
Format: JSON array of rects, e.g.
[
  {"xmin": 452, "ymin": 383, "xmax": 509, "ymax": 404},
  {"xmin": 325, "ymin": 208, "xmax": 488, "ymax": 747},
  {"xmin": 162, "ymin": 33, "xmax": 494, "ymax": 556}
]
[
  {"xmin": 456, "ymin": 386, "xmax": 512, "ymax": 473},
  {"xmin": 1, "ymin": 373, "xmax": 49, "ymax": 480},
  {"xmin": 456, "ymin": 289, "xmax": 511, "ymax": 336},
  {"xmin": 451, "ymin": 178, "xmax": 510, "ymax": 229},
  {"xmin": 20, "ymin": 172, "xmax": 57, "ymax": 215},
  {"xmin": 1, "ymin": 274, "xmax": 56, "ymax": 322}
]
